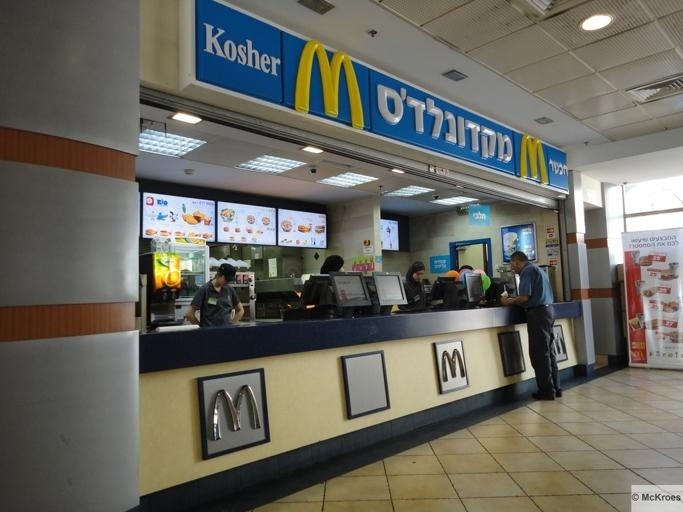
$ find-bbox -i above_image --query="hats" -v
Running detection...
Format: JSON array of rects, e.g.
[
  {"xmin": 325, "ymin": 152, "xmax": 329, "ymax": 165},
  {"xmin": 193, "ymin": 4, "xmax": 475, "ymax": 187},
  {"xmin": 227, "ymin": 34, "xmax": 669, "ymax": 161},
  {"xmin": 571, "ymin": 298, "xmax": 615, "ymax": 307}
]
[
  {"xmin": 217, "ymin": 263, "xmax": 236, "ymax": 282},
  {"xmin": 318, "ymin": 255, "xmax": 344, "ymax": 274}
]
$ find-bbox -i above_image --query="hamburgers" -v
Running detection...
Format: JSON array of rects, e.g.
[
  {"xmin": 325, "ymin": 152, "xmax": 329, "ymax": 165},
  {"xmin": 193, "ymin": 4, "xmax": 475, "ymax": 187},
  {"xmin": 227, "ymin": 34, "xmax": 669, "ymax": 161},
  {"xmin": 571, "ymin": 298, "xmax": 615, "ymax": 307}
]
[{"xmin": 639, "ymin": 256, "xmax": 652, "ymax": 266}]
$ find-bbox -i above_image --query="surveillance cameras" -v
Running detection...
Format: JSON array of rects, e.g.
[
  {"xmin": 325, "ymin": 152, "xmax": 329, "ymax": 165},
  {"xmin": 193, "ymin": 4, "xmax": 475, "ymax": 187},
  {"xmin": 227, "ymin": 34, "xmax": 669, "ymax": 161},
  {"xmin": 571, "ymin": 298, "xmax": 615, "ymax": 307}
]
[
  {"xmin": 308, "ymin": 166, "xmax": 318, "ymax": 176},
  {"xmin": 433, "ymin": 195, "xmax": 439, "ymax": 199}
]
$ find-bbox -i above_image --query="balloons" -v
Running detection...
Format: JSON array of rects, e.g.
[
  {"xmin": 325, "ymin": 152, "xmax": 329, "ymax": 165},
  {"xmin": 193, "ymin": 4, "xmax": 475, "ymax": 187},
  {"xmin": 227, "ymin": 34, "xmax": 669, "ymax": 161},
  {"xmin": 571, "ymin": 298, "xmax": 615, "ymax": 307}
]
[{"xmin": 447, "ymin": 269, "xmax": 490, "ymax": 290}]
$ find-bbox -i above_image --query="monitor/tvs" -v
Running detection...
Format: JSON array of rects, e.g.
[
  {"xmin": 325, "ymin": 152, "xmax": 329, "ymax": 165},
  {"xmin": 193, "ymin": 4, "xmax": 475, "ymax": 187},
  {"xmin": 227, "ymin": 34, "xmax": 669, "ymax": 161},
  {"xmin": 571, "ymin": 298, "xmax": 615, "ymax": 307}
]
[
  {"xmin": 371, "ymin": 270, "xmax": 409, "ymax": 306},
  {"xmin": 299, "ymin": 274, "xmax": 336, "ymax": 307},
  {"xmin": 429, "ymin": 276, "xmax": 457, "ymax": 312},
  {"xmin": 464, "ymin": 272, "xmax": 486, "ymax": 302},
  {"xmin": 330, "ymin": 270, "xmax": 373, "ymax": 308}
]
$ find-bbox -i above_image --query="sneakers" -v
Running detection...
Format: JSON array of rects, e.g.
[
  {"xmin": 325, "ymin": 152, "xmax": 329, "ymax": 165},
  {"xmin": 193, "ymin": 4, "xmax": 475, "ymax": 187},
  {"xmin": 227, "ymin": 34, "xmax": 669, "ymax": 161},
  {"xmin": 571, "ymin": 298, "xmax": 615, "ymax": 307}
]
[{"xmin": 530, "ymin": 389, "xmax": 562, "ymax": 402}]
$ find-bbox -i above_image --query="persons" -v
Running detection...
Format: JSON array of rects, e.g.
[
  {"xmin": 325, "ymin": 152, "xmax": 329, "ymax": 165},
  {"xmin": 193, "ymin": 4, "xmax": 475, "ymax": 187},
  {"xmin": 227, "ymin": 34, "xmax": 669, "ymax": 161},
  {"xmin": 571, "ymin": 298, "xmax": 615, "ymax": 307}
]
[
  {"xmin": 186, "ymin": 263, "xmax": 244, "ymax": 325},
  {"xmin": 397, "ymin": 260, "xmax": 427, "ymax": 309},
  {"xmin": 500, "ymin": 251, "xmax": 562, "ymax": 400}
]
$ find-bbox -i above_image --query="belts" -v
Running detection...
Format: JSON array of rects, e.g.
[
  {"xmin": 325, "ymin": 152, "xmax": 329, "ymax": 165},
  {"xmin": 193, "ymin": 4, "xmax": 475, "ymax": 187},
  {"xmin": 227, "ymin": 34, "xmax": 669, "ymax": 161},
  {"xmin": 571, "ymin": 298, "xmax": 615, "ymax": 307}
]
[{"xmin": 524, "ymin": 304, "xmax": 550, "ymax": 309}]
